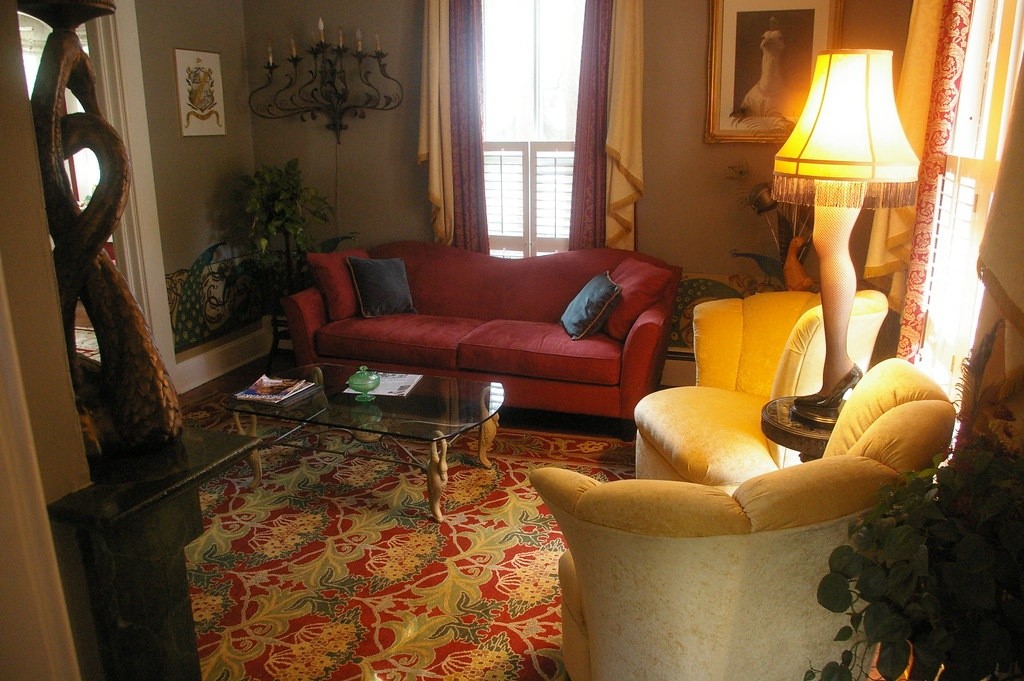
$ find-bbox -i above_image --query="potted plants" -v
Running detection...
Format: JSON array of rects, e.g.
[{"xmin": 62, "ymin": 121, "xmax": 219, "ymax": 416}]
[{"xmin": 225, "ymin": 155, "xmax": 358, "ymax": 311}]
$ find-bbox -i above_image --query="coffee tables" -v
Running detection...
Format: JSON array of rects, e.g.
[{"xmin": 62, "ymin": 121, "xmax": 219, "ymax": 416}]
[{"xmin": 225, "ymin": 362, "xmax": 505, "ymax": 525}]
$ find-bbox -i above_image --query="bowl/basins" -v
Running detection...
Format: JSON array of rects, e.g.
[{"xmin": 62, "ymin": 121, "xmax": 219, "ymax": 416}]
[{"xmin": 348, "ymin": 365, "xmax": 380, "ymax": 402}]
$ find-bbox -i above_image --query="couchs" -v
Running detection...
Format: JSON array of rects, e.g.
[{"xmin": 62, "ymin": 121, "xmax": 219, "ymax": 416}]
[
  {"xmin": 528, "ymin": 357, "xmax": 956, "ymax": 681},
  {"xmin": 631, "ymin": 288, "xmax": 889, "ymax": 490},
  {"xmin": 285, "ymin": 239, "xmax": 683, "ymax": 441}
]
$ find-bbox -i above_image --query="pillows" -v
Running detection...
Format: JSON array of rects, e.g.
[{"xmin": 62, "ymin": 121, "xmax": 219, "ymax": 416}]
[
  {"xmin": 305, "ymin": 247, "xmax": 371, "ymax": 322},
  {"xmin": 558, "ymin": 270, "xmax": 623, "ymax": 341},
  {"xmin": 601, "ymin": 257, "xmax": 673, "ymax": 343},
  {"xmin": 346, "ymin": 256, "xmax": 418, "ymax": 319}
]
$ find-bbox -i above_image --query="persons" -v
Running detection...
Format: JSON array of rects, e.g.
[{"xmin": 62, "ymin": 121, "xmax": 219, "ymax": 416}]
[{"xmin": 796, "ymin": 180, "xmax": 868, "ymax": 412}]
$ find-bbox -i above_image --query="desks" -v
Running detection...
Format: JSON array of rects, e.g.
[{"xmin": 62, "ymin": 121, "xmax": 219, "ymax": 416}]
[
  {"xmin": 267, "ymin": 306, "xmax": 296, "ymax": 371},
  {"xmin": 44, "ymin": 426, "xmax": 263, "ymax": 681}
]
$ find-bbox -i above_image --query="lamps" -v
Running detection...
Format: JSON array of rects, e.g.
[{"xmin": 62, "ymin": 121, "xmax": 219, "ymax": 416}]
[
  {"xmin": 247, "ymin": 16, "xmax": 404, "ymax": 146},
  {"xmin": 771, "ymin": 49, "xmax": 921, "ymax": 428}
]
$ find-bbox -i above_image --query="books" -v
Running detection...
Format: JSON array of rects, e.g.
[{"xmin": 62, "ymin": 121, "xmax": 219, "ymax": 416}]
[
  {"xmin": 233, "ymin": 383, "xmax": 315, "ymax": 404},
  {"xmin": 237, "ymin": 374, "xmax": 305, "ymax": 401}
]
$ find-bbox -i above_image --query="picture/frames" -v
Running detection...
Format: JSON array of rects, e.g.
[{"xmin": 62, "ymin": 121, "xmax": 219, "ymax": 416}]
[
  {"xmin": 704, "ymin": 0, "xmax": 844, "ymax": 144},
  {"xmin": 172, "ymin": 46, "xmax": 228, "ymax": 138}
]
